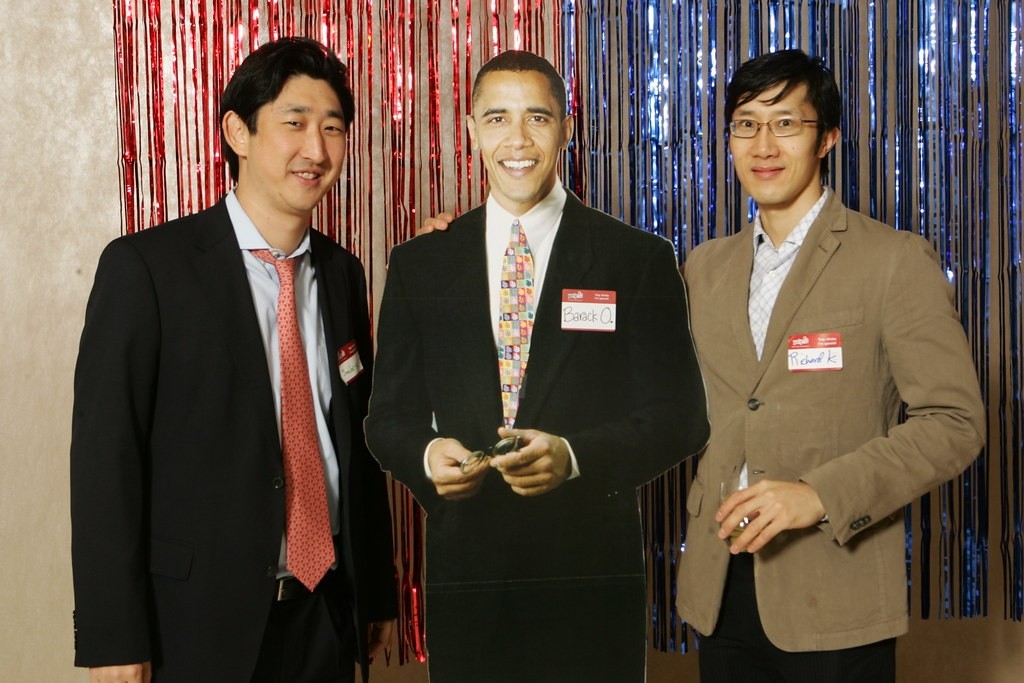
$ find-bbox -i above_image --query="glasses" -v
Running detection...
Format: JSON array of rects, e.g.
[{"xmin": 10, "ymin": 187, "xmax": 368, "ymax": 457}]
[
  {"xmin": 460, "ymin": 435, "xmax": 527, "ymax": 474},
  {"xmin": 728, "ymin": 116, "xmax": 824, "ymax": 138}
]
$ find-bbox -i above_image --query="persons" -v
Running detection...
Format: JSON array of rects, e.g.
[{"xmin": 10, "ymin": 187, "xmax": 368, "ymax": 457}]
[
  {"xmin": 680, "ymin": 47, "xmax": 986, "ymax": 682},
  {"xmin": 72, "ymin": 34, "xmax": 401, "ymax": 682},
  {"xmin": 367, "ymin": 51, "xmax": 711, "ymax": 683}
]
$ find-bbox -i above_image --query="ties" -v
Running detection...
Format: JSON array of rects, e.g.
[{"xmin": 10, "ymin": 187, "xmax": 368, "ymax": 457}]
[
  {"xmin": 250, "ymin": 249, "xmax": 337, "ymax": 591},
  {"xmin": 498, "ymin": 219, "xmax": 537, "ymax": 430}
]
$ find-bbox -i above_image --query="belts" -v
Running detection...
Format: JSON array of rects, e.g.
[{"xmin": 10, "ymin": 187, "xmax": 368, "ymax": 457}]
[{"xmin": 271, "ymin": 568, "xmax": 337, "ymax": 601}]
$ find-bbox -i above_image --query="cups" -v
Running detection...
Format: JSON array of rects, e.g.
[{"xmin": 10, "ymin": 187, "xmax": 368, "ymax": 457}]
[{"xmin": 721, "ymin": 473, "xmax": 772, "ymax": 551}]
